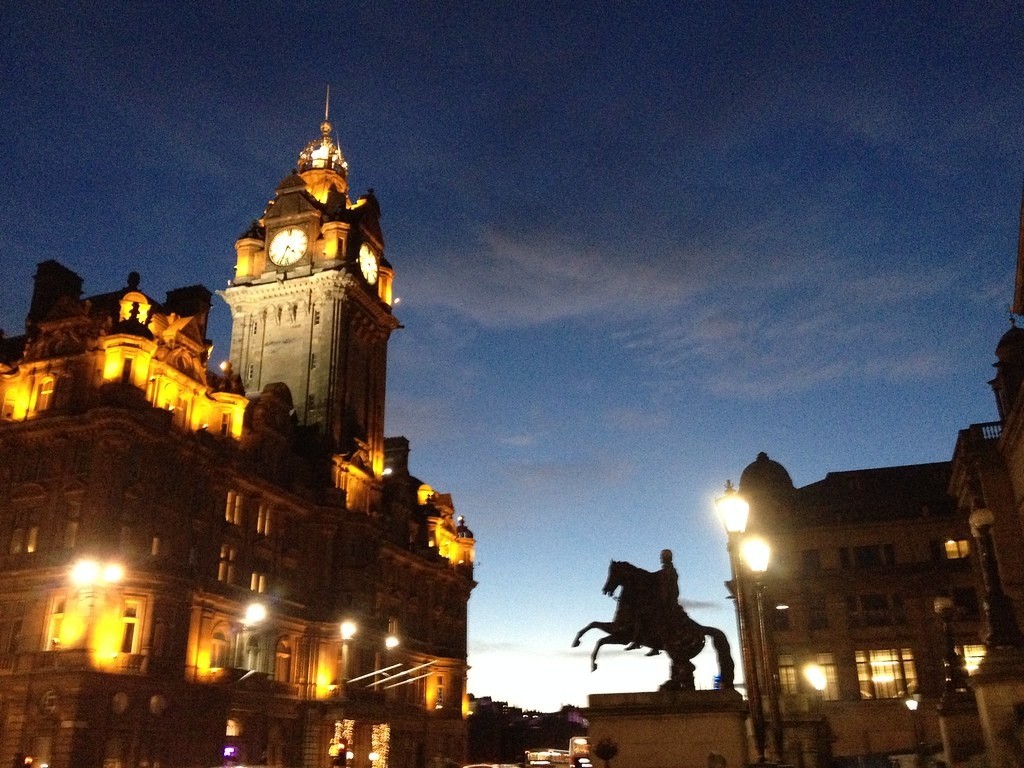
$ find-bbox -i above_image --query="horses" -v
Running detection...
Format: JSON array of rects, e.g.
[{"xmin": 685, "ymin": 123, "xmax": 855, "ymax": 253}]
[{"xmin": 572, "ymin": 560, "xmax": 734, "ymax": 691}]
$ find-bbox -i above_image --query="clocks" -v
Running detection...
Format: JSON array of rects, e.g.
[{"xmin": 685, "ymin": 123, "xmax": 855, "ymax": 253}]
[
  {"xmin": 360, "ymin": 246, "xmax": 378, "ymax": 285},
  {"xmin": 269, "ymin": 229, "xmax": 308, "ymax": 265}
]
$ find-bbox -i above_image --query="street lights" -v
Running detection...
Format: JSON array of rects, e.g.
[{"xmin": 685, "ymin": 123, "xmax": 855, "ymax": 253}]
[
  {"xmin": 714, "ymin": 480, "xmax": 767, "ymax": 768},
  {"xmin": 737, "ymin": 530, "xmax": 786, "ymax": 768},
  {"xmin": 933, "ymin": 595, "xmax": 983, "ymax": 716},
  {"xmin": 969, "ymin": 509, "xmax": 1024, "ymax": 649}
]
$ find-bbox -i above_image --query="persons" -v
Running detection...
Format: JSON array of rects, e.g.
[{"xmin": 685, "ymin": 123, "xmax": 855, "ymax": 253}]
[{"xmin": 623, "ymin": 548, "xmax": 679, "ymax": 656}]
